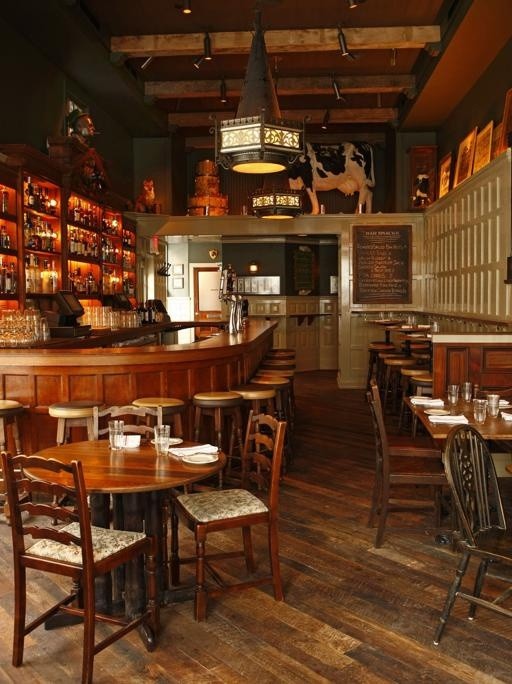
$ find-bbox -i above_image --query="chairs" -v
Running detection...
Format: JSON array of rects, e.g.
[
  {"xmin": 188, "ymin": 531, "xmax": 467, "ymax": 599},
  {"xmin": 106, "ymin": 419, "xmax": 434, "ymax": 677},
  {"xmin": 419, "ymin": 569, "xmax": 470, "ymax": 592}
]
[
  {"xmin": 366, "ymin": 393, "xmax": 460, "ymax": 548},
  {"xmin": 1, "ymin": 451, "xmax": 160, "ymax": 683},
  {"xmin": 168, "ymin": 411, "xmax": 284, "ymax": 626},
  {"xmin": 434, "ymin": 425, "xmax": 510, "ymax": 647},
  {"xmin": 370, "ymin": 378, "xmax": 443, "ymax": 523},
  {"xmin": 93, "ymin": 403, "xmax": 182, "ymax": 584}
]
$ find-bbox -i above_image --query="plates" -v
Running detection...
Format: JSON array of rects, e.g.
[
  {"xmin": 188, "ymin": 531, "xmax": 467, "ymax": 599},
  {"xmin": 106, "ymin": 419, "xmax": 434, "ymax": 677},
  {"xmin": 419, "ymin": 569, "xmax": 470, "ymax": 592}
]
[
  {"xmin": 109, "ymin": 436, "xmax": 143, "ymax": 449},
  {"xmin": 150, "ymin": 436, "xmax": 183, "ymax": 448},
  {"xmin": 182, "ymin": 452, "xmax": 219, "ymax": 465}
]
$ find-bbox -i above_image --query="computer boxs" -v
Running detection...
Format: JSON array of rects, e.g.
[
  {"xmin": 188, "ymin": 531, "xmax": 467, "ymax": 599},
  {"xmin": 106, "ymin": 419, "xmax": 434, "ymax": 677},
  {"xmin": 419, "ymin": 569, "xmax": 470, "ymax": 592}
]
[{"xmin": 49, "ymin": 325, "xmax": 91, "ymax": 337}]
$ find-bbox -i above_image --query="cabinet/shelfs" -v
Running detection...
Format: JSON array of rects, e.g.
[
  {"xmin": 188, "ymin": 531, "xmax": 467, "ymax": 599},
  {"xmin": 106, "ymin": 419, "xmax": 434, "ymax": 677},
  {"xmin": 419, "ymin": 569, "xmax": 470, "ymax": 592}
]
[{"xmin": 0, "ymin": 166, "xmax": 135, "ymax": 305}]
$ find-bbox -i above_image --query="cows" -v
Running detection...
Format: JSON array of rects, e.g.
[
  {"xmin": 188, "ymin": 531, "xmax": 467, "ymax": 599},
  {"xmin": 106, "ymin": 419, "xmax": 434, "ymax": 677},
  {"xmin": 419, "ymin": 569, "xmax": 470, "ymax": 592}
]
[{"xmin": 287, "ymin": 140, "xmax": 375, "ymax": 215}]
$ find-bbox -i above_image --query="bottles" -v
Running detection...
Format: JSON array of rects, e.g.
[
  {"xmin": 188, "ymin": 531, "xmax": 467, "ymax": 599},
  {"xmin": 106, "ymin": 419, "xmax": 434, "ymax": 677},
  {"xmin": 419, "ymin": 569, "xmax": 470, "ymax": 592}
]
[{"xmin": 0, "ymin": 176, "xmax": 139, "ymax": 298}]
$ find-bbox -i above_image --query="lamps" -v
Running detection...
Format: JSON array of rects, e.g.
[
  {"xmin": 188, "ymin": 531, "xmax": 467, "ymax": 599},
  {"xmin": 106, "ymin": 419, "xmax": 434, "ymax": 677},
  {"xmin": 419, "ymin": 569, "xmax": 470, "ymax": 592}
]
[
  {"xmin": 141, "ymin": 9, "xmax": 398, "ymax": 219},
  {"xmin": 249, "ymin": 261, "xmax": 259, "ymax": 274}
]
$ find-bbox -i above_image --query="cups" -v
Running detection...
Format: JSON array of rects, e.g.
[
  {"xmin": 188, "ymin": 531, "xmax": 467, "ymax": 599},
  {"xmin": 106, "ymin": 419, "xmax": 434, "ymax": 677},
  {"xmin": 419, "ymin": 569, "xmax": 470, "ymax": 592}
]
[
  {"xmin": 378, "ymin": 308, "xmax": 441, "ymax": 333},
  {"xmin": 106, "ymin": 419, "xmax": 124, "ymax": 452},
  {"xmin": 108, "ymin": 451, "xmax": 126, "ymax": 482},
  {"xmin": 1, "ymin": 305, "xmax": 53, "ymax": 348},
  {"xmin": 79, "ymin": 298, "xmax": 172, "ymax": 334},
  {"xmin": 152, "ymin": 425, "xmax": 169, "ymax": 455},
  {"xmin": 156, "ymin": 457, "xmax": 170, "ymax": 482},
  {"xmin": 448, "ymin": 381, "xmax": 510, "ymax": 426}
]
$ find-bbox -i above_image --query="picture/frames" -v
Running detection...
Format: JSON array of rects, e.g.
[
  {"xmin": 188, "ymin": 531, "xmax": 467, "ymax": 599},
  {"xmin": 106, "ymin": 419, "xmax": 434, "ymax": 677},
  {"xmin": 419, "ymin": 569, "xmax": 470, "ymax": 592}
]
[
  {"xmin": 172, "ymin": 264, "xmax": 184, "ymax": 276},
  {"xmin": 435, "ymin": 87, "xmax": 512, "ymax": 202},
  {"xmin": 172, "ymin": 278, "xmax": 184, "ymax": 289}
]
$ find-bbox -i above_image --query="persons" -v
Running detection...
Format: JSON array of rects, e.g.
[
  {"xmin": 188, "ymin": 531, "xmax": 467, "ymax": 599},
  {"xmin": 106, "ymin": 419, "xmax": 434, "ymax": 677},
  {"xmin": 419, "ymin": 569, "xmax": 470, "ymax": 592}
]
[{"xmin": 66, "ymin": 108, "xmax": 112, "ymax": 187}]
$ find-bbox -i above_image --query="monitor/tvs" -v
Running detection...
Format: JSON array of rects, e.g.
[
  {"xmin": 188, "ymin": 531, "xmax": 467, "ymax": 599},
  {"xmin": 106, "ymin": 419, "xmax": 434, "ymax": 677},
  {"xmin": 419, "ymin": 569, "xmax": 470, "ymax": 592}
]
[
  {"xmin": 54, "ymin": 291, "xmax": 85, "ymax": 327},
  {"xmin": 112, "ymin": 293, "xmax": 131, "ymax": 308}
]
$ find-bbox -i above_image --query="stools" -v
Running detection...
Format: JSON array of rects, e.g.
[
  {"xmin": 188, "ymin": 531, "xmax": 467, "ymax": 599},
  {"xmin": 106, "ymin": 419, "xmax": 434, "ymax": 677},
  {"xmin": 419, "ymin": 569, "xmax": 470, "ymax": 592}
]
[
  {"xmin": 251, "ymin": 349, "xmax": 295, "ymax": 474},
  {"xmin": 232, "ymin": 386, "xmax": 285, "ymax": 493},
  {"xmin": 366, "ymin": 340, "xmax": 436, "ymax": 439},
  {"xmin": 48, "ymin": 402, "xmax": 105, "ymax": 524},
  {"xmin": 189, "ymin": 390, "xmax": 251, "ymax": 491},
  {"xmin": 133, "ymin": 397, "xmax": 193, "ymax": 519},
  {"xmin": 1, "ymin": 401, "xmax": 33, "ymax": 526}
]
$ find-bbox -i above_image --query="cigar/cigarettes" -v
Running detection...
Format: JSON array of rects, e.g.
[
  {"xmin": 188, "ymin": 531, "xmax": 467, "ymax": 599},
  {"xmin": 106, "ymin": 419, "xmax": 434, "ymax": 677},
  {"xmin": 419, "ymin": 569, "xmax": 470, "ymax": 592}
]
[{"xmin": 94, "ymin": 131, "xmax": 104, "ymax": 135}]
